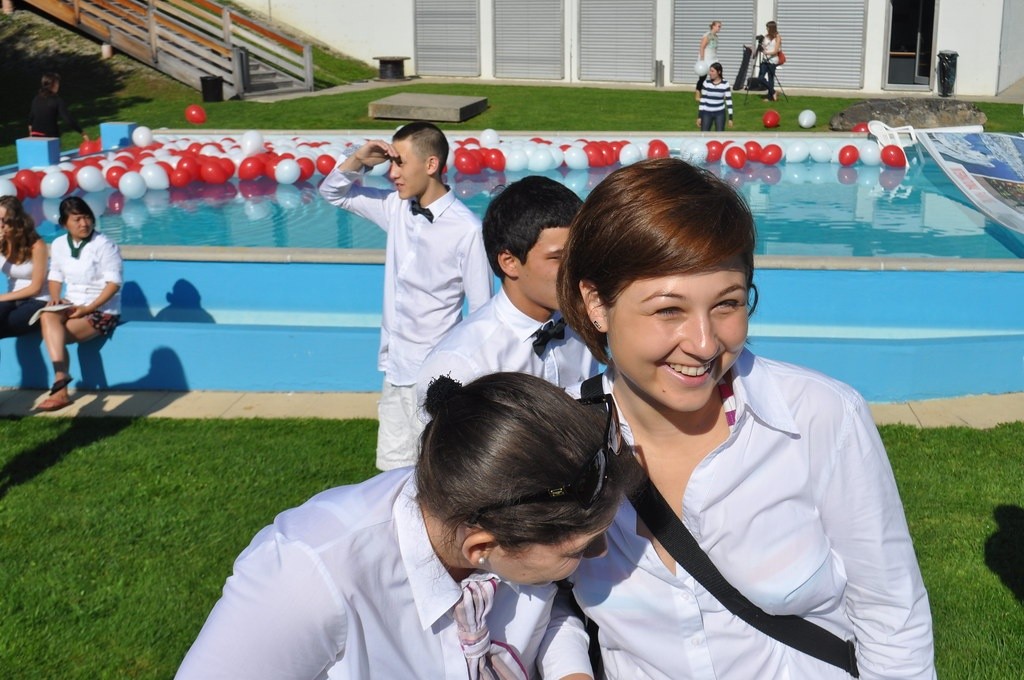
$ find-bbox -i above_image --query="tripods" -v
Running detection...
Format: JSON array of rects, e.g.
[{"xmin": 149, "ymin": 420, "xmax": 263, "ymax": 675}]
[{"xmin": 744, "ymin": 43, "xmax": 789, "ymax": 104}]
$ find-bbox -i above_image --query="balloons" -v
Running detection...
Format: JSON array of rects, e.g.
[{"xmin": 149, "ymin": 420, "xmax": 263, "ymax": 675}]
[
  {"xmin": 703, "ymin": 162, "xmax": 905, "ymax": 189},
  {"xmin": 186, "ymin": 105, "xmax": 206, "ymax": 124},
  {"xmin": 680, "ymin": 139, "xmax": 907, "ymax": 168},
  {"xmin": 798, "ymin": 109, "xmax": 816, "ymax": 128},
  {"xmin": 762, "ymin": 110, "xmax": 780, "ymax": 127},
  {"xmin": 851, "ymin": 123, "xmax": 869, "ymax": 132},
  {"xmin": 0, "ymin": 126, "xmax": 671, "ymax": 200},
  {"xmin": 694, "ymin": 62, "xmax": 709, "ymax": 77},
  {"xmin": 22, "ymin": 177, "xmax": 520, "ymax": 232}
]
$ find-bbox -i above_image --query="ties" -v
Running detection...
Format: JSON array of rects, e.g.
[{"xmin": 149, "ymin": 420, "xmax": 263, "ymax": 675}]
[{"xmin": 448, "ymin": 579, "xmax": 529, "ymax": 680}]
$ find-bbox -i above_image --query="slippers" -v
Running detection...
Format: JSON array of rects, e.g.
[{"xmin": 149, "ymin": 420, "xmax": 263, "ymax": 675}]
[
  {"xmin": 49, "ymin": 376, "xmax": 73, "ymax": 394},
  {"xmin": 39, "ymin": 391, "xmax": 71, "ymax": 410}
]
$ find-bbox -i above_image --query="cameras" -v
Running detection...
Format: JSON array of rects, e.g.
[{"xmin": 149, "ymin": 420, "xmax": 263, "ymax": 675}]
[{"xmin": 756, "ymin": 35, "xmax": 764, "ymax": 43}]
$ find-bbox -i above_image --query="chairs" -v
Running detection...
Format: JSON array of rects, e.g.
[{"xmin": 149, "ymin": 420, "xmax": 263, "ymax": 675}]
[
  {"xmin": 868, "ymin": 157, "xmax": 917, "ymax": 200},
  {"xmin": 868, "ymin": 119, "xmax": 923, "ymax": 168}
]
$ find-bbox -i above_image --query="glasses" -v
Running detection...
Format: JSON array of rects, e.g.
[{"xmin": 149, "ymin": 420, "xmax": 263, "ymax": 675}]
[{"xmin": 467, "ymin": 393, "xmax": 622, "ymax": 528}]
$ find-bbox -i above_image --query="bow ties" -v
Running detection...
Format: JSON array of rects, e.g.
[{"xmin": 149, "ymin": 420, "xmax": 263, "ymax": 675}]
[
  {"xmin": 411, "ymin": 199, "xmax": 434, "ymax": 224},
  {"xmin": 533, "ymin": 316, "xmax": 567, "ymax": 357}
]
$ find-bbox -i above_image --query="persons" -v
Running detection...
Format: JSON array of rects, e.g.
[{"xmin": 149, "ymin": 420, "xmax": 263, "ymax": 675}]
[
  {"xmin": 534, "ymin": 157, "xmax": 937, "ymax": 680},
  {"xmin": 696, "ymin": 63, "xmax": 733, "ymax": 131},
  {"xmin": 0, "ymin": 195, "xmax": 50, "ymax": 340},
  {"xmin": 416, "ymin": 176, "xmax": 599, "ymax": 425},
  {"xmin": 695, "ymin": 20, "xmax": 723, "ymax": 100},
  {"xmin": 318, "ymin": 122, "xmax": 494, "ymax": 471},
  {"xmin": 27, "ymin": 73, "xmax": 88, "ymax": 143},
  {"xmin": 173, "ymin": 368, "xmax": 638, "ymax": 680},
  {"xmin": 39, "ymin": 197, "xmax": 124, "ymax": 410},
  {"xmin": 758, "ymin": 21, "xmax": 780, "ymax": 102}
]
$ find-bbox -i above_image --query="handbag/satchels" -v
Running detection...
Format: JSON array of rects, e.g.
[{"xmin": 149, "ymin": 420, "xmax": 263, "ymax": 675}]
[{"xmin": 779, "ymin": 52, "xmax": 787, "ymax": 65}]
